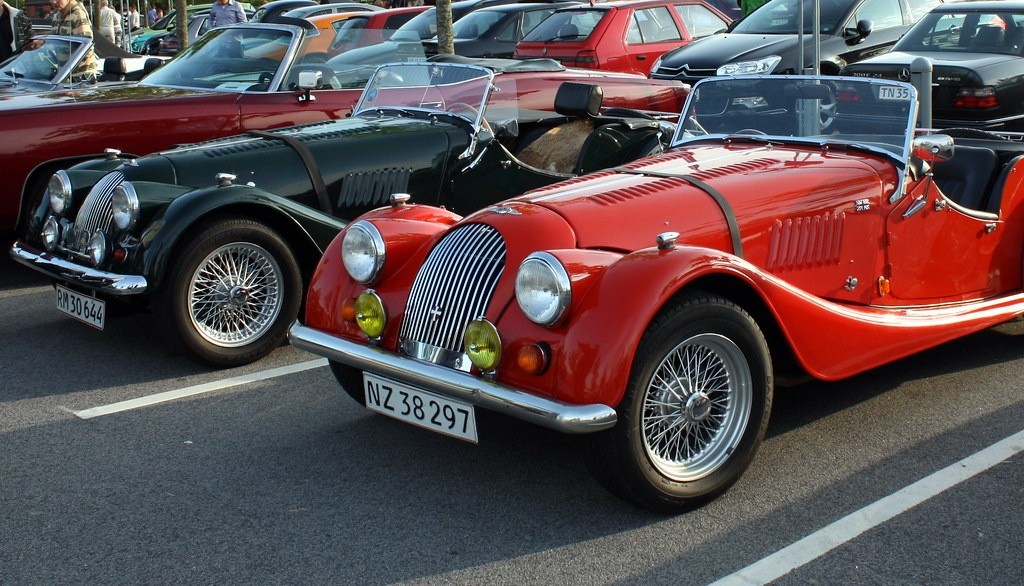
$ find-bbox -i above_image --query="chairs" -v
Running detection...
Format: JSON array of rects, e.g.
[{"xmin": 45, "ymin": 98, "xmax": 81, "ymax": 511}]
[
  {"xmin": 142, "ymin": 57, "xmax": 165, "ymax": 80},
  {"xmin": 514, "ymin": 82, "xmax": 605, "ymax": 173},
  {"xmin": 558, "ymin": 24, "xmax": 580, "ymax": 44},
  {"xmin": 1007, "ymin": 27, "xmax": 1023, "ymax": 49},
  {"xmin": 976, "ymin": 25, "xmax": 1006, "ymax": 45},
  {"xmin": 98, "ymin": 57, "xmax": 127, "ymax": 84}
]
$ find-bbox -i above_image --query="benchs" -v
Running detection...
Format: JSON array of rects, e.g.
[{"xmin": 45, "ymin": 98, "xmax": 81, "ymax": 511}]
[{"xmin": 798, "ymin": 136, "xmax": 998, "ymax": 206}]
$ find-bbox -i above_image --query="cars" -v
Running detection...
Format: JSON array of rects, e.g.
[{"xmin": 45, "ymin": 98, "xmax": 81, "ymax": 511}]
[
  {"xmin": 0, "ymin": 21, "xmax": 696, "ymax": 232},
  {"xmin": 513, "ymin": 0, "xmax": 734, "ymax": 81},
  {"xmin": 124, "ymin": 2, "xmax": 385, "ymax": 64},
  {"xmin": 649, "ymin": 0, "xmax": 1024, "ymax": 136},
  {"xmin": 833, "ymin": 2, "xmax": 1024, "ymax": 141},
  {"xmin": 0, "ymin": 34, "xmax": 165, "ymax": 96},
  {"xmin": 287, "ymin": 75, "xmax": 1023, "ymax": 512},
  {"xmin": 315, "ymin": 0, "xmax": 562, "ymax": 89},
  {"xmin": 394, "ymin": 4, "xmax": 586, "ymax": 85},
  {"xmin": 10, "ymin": 62, "xmax": 696, "ymax": 366}
]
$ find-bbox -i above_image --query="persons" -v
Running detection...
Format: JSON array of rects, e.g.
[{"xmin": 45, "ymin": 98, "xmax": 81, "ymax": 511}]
[
  {"xmin": 100, "ymin": 0, "xmax": 139, "ymax": 44},
  {"xmin": 206, "ymin": 0, "xmax": 248, "ymax": 59},
  {"xmin": 148, "ymin": 3, "xmax": 163, "ymax": 27},
  {"xmin": 0, "ymin": 0, "xmax": 98, "ymax": 82}
]
